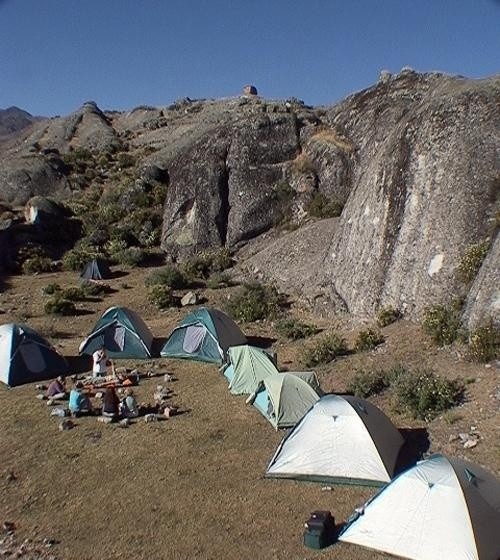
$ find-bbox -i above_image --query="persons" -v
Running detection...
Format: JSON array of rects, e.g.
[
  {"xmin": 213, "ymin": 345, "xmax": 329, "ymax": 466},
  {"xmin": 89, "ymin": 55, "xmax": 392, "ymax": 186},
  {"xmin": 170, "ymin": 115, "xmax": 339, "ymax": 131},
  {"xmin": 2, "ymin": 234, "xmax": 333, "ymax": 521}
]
[
  {"xmin": 119, "ymin": 387, "xmax": 139, "ymax": 418},
  {"xmin": 69, "ymin": 381, "xmax": 94, "ymax": 415},
  {"xmin": 45, "ymin": 375, "xmax": 69, "ymax": 401},
  {"xmin": 102, "ymin": 386, "xmax": 121, "ymax": 417},
  {"xmin": 92, "ymin": 344, "xmax": 113, "ymax": 378}
]
